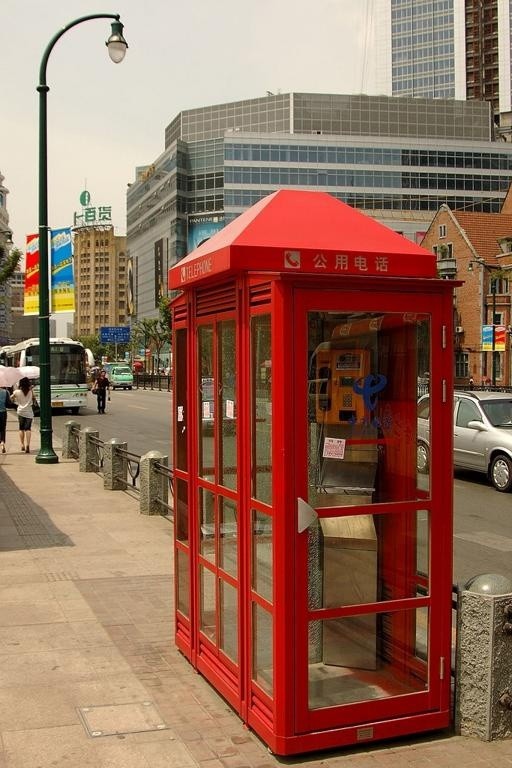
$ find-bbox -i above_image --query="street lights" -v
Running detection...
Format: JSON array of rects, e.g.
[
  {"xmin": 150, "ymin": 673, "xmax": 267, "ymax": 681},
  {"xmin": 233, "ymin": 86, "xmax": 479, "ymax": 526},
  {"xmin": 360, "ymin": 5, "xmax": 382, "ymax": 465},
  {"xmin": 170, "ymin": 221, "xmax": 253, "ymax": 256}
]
[
  {"xmin": 36, "ymin": 13, "xmax": 129, "ymax": 463},
  {"xmin": 468, "ymin": 260, "xmax": 496, "ymax": 388}
]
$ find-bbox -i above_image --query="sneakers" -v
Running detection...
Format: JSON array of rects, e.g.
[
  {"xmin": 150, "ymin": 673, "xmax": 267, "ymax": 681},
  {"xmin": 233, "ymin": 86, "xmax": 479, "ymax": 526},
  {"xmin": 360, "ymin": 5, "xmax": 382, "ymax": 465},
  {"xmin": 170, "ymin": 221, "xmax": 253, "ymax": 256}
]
[
  {"xmin": 21, "ymin": 445, "xmax": 30, "ymax": 453},
  {"xmin": 98, "ymin": 409, "xmax": 105, "ymax": 414}
]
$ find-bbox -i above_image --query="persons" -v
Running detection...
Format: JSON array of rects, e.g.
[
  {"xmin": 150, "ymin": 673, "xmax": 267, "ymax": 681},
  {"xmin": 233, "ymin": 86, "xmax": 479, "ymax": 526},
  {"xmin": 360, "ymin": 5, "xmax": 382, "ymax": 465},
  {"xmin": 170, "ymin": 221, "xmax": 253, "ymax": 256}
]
[
  {"xmin": 94, "ymin": 371, "xmax": 110, "ymax": 414},
  {"xmin": 0, "ymin": 377, "xmax": 40, "ymax": 454}
]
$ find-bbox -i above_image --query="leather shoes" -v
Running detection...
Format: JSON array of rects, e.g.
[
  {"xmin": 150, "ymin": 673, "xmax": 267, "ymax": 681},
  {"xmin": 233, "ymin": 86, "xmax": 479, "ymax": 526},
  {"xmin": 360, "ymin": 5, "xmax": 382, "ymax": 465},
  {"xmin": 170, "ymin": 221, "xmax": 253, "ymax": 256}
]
[{"xmin": 1, "ymin": 443, "xmax": 5, "ymax": 453}]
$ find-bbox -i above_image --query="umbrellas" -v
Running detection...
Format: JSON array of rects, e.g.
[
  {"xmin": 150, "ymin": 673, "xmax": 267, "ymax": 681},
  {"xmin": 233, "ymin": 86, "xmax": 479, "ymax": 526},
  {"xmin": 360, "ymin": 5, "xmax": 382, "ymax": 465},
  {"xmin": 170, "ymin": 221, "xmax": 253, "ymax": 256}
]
[{"xmin": 0, "ymin": 365, "xmax": 40, "ymax": 388}]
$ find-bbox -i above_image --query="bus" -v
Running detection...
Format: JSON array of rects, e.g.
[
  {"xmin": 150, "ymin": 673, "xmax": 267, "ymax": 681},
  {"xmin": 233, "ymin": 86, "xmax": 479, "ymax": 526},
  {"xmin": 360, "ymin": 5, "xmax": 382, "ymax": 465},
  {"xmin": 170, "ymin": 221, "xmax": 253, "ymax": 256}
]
[{"xmin": 0, "ymin": 336, "xmax": 95, "ymax": 417}]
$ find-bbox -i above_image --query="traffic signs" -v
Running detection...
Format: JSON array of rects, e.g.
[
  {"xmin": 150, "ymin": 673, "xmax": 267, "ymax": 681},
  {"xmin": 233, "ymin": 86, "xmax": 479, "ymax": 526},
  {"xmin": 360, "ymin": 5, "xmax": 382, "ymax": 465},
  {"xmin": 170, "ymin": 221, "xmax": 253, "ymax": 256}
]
[{"xmin": 100, "ymin": 326, "xmax": 129, "ymax": 343}]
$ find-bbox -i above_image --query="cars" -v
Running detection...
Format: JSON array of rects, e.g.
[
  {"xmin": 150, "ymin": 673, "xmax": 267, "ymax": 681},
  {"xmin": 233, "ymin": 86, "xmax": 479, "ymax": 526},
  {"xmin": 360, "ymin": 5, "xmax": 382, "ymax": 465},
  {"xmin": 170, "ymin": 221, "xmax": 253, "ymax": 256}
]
[
  {"xmin": 199, "ymin": 378, "xmax": 236, "ymax": 438},
  {"xmin": 417, "ymin": 390, "xmax": 512, "ymax": 492}
]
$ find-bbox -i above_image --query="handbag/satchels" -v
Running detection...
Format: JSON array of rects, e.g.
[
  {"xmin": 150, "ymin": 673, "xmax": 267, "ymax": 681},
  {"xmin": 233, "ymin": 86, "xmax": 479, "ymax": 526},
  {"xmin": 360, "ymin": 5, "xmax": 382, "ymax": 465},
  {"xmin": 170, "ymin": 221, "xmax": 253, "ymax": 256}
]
[
  {"xmin": 31, "ymin": 400, "xmax": 40, "ymax": 417},
  {"xmin": 5, "ymin": 391, "xmax": 13, "ymax": 408}
]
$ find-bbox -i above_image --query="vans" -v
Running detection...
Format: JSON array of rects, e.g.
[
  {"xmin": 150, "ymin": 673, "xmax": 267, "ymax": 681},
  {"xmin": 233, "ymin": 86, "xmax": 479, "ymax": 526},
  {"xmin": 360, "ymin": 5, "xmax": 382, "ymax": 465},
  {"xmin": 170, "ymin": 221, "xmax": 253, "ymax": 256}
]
[{"xmin": 109, "ymin": 366, "xmax": 134, "ymax": 390}]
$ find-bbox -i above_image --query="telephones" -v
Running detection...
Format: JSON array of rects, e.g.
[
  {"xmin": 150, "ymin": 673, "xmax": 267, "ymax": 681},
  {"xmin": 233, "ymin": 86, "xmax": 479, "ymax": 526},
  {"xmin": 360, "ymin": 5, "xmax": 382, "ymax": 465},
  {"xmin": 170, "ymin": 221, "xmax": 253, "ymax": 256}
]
[{"xmin": 315, "ymin": 349, "xmax": 370, "ymax": 425}]
